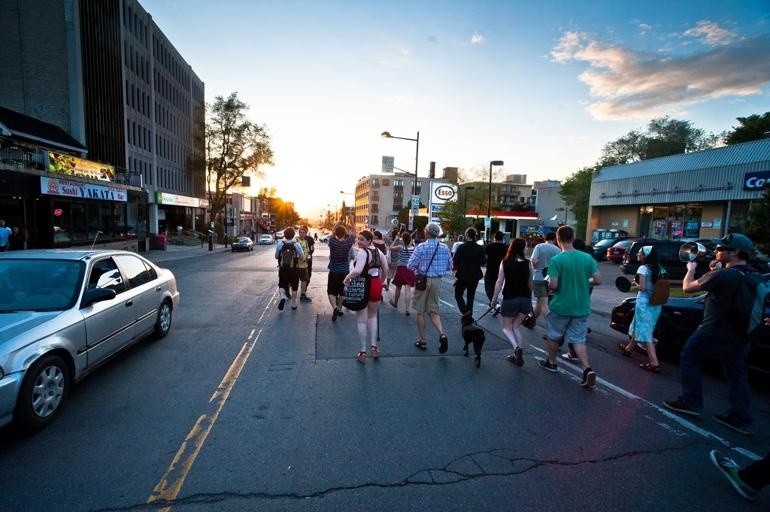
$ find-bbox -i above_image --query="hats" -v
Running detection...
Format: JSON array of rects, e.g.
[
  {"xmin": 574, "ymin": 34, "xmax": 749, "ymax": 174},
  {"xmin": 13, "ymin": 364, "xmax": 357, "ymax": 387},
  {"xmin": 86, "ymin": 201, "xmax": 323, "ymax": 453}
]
[{"xmin": 712, "ymin": 232, "xmax": 755, "ymax": 253}]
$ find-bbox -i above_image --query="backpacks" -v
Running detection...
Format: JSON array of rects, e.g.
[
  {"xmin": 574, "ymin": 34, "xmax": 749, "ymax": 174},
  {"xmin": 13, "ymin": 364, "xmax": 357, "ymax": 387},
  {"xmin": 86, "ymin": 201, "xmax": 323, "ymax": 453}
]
[
  {"xmin": 726, "ymin": 266, "xmax": 770, "ymax": 340},
  {"xmin": 277, "ymin": 241, "xmax": 298, "ymax": 270},
  {"xmin": 646, "ymin": 264, "xmax": 671, "ymax": 305}
]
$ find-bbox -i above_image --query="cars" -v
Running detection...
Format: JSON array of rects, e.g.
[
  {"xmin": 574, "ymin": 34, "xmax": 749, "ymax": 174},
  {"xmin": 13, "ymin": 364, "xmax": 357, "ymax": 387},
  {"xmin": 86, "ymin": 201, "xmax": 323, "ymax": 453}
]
[
  {"xmin": 0, "ymin": 248, "xmax": 182, "ymax": 424},
  {"xmin": 294, "ymin": 225, "xmax": 299, "ymax": 229},
  {"xmin": 593, "ymin": 239, "xmax": 616, "ymax": 260},
  {"xmin": 283, "ymin": 231, "xmax": 285, "ymax": 236},
  {"xmin": 231, "ymin": 237, "xmax": 253, "ymax": 250},
  {"xmin": 623, "ymin": 239, "xmax": 711, "ymax": 279},
  {"xmin": 276, "ymin": 232, "xmax": 283, "ymax": 238},
  {"xmin": 696, "ymin": 238, "xmax": 769, "ymax": 268},
  {"xmin": 319, "ymin": 235, "xmax": 331, "ymax": 242},
  {"xmin": 258, "ymin": 234, "xmax": 273, "ymax": 244},
  {"xmin": 609, "ymin": 272, "xmax": 770, "ymax": 347},
  {"xmin": 607, "ymin": 239, "xmax": 641, "ymax": 263}
]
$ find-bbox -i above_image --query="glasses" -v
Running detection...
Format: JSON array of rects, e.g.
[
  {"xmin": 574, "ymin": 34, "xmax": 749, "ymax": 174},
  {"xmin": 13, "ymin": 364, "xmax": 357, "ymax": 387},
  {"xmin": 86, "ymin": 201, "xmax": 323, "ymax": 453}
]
[{"xmin": 713, "ymin": 247, "xmax": 726, "ymax": 251}]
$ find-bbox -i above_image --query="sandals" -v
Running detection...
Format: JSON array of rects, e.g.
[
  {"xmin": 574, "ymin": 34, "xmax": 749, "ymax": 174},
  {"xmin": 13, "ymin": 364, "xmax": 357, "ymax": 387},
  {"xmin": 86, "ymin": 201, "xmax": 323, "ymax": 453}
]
[
  {"xmin": 357, "ymin": 351, "xmax": 367, "ymax": 362},
  {"xmin": 370, "ymin": 346, "xmax": 381, "ymax": 357},
  {"xmin": 438, "ymin": 334, "xmax": 448, "ymax": 354},
  {"xmin": 415, "ymin": 341, "xmax": 426, "ymax": 351}
]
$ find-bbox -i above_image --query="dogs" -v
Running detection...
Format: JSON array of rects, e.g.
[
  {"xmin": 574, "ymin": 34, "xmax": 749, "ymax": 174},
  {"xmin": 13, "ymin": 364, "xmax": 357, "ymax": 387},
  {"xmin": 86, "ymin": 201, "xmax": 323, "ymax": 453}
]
[{"xmin": 461, "ymin": 310, "xmax": 485, "ymax": 368}]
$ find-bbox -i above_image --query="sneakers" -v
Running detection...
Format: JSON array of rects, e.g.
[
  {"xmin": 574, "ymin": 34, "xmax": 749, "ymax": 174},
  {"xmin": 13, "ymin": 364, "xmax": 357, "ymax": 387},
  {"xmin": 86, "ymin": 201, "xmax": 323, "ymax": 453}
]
[
  {"xmin": 710, "ymin": 450, "xmax": 760, "ymax": 500},
  {"xmin": 278, "ymin": 296, "xmax": 311, "ymax": 310},
  {"xmin": 663, "ymin": 395, "xmax": 702, "ymax": 416},
  {"xmin": 332, "ymin": 306, "xmax": 340, "ymax": 321},
  {"xmin": 639, "ymin": 362, "xmax": 661, "ymax": 373},
  {"xmin": 506, "ymin": 346, "xmax": 595, "ymax": 389},
  {"xmin": 712, "ymin": 408, "xmax": 755, "ymax": 434},
  {"xmin": 619, "ymin": 342, "xmax": 632, "ymax": 356}
]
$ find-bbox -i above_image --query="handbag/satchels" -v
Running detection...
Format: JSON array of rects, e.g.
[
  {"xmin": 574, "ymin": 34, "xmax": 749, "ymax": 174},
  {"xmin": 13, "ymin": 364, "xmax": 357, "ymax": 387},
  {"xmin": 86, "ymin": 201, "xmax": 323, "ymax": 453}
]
[
  {"xmin": 521, "ymin": 308, "xmax": 536, "ymax": 331},
  {"xmin": 342, "ymin": 248, "xmax": 371, "ymax": 311},
  {"xmin": 415, "ymin": 274, "xmax": 427, "ymax": 290}
]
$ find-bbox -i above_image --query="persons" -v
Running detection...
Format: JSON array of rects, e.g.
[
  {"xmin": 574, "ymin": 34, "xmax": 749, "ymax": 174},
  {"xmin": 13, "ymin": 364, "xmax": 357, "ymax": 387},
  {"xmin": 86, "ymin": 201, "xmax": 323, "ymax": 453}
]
[
  {"xmin": 661, "ymin": 233, "xmax": 759, "ymax": 436},
  {"xmin": 176, "ymin": 223, "xmax": 183, "ymax": 232},
  {"xmin": 710, "ymin": 449, "xmax": 770, "ymax": 501},
  {"xmin": 328, "ymin": 222, "xmax": 454, "ymax": 359},
  {"xmin": 276, "ymin": 225, "xmax": 314, "ymax": 311},
  {"xmin": 450, "ymin": 225, "xmax": 603, "ymax": 388},
  {"xmin": 0, "ymin": 219, "xmax": 13, "ymax": 252},
  {"xmin": 619, "ymin": 244, "xmax": 668, "ymax": 374},
  {"xmin": 6, "ymin": 226, "xmax": 22, "ymax": 250}
]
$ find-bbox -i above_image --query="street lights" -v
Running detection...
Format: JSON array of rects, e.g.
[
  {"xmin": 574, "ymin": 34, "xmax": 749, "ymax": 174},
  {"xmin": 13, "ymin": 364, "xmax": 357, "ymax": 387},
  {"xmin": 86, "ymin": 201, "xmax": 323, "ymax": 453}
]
[
  {"xmin": 489, "ymin": 158, "xmax": 504, "ymax": 214},
  {"xmin": 381, "ymin": 128, "xmax": 419, "ymax": 198},
  {"xmin": 339, "ymin": 189, "xmax": 355, "ymax": 196},
  {"xmin": 327, "ymin": 204, "xmax": 337, "ymax": 221},
  {"xmin": 323, "ymin": 209, "xmax": 325, "ymax": 211}
]
